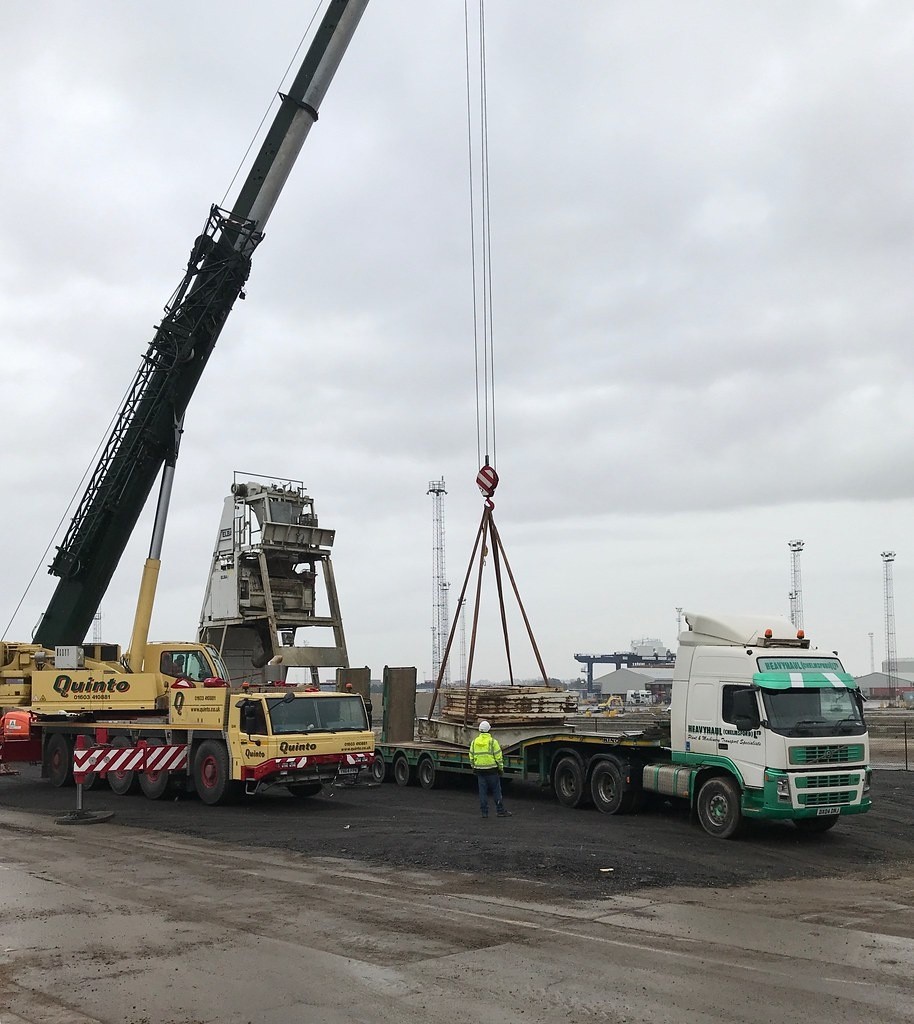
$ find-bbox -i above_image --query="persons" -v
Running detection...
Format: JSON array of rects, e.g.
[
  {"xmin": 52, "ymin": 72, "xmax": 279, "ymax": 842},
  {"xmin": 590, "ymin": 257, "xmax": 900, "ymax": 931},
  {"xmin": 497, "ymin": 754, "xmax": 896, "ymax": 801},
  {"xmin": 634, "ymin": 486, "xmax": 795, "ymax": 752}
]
[
  {"xmin": 666, "ymin": 649, "xmax": 673, "ymax": 661},
  {"xmin": 469, "ymin": 721, "xmax": 512, "ymax": 818},
  {"xmin": 652, "ymin": 648, "xmax": 658, "ymax": 660},
  {"xmin": 171, "ymin": 654, "xmax": 186, "ymax": 677}
]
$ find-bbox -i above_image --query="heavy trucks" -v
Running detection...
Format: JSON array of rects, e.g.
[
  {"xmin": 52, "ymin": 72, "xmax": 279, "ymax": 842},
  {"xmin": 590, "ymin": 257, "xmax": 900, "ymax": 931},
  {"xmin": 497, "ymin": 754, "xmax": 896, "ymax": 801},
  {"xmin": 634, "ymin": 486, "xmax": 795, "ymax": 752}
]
[
  {"xmin": 371, "ymin": 606, "xmax": 875, "ymax": 839},
  {"xmin": 29, "ymin": 686, "xmax": 376, "ymax": 807}
]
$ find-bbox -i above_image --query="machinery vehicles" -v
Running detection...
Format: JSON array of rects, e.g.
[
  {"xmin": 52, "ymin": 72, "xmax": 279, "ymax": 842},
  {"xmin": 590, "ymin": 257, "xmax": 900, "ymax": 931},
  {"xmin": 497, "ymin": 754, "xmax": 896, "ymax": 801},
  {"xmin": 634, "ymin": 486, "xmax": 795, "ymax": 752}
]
[{"xmin": 0, "ymin": 1, "xmax": 501, "ymax": 721}]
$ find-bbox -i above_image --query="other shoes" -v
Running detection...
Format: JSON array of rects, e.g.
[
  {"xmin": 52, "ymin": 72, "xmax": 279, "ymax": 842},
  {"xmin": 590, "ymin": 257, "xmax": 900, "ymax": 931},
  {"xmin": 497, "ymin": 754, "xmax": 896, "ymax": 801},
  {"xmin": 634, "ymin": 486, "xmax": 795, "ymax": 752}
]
[
  {"xmin": 497, "ymin": 811, "xmax": 512, "ymax": 817},
  {"xmin": 482, "ymin": 814, "xmax": 488, "ymax": 818}
]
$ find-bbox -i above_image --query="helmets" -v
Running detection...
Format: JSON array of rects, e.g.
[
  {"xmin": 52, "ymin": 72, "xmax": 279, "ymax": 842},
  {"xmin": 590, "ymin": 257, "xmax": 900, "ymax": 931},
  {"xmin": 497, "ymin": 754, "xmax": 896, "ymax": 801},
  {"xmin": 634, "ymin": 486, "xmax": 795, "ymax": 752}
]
[{"xmin": 479, "ymin": 721, "xmax": 491, "ymax": 732}]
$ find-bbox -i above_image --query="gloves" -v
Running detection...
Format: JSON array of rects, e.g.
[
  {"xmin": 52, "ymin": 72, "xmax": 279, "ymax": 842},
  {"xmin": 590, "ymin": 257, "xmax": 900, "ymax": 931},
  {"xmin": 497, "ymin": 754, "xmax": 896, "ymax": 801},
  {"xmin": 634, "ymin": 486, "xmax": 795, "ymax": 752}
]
[
  {"xmin": 498, "ymin": 770, "xmax": 504, "ymax": 776},
  {"xmin": 473, "ymin": 769, "xmax": 477, "ymax": 775}
]
